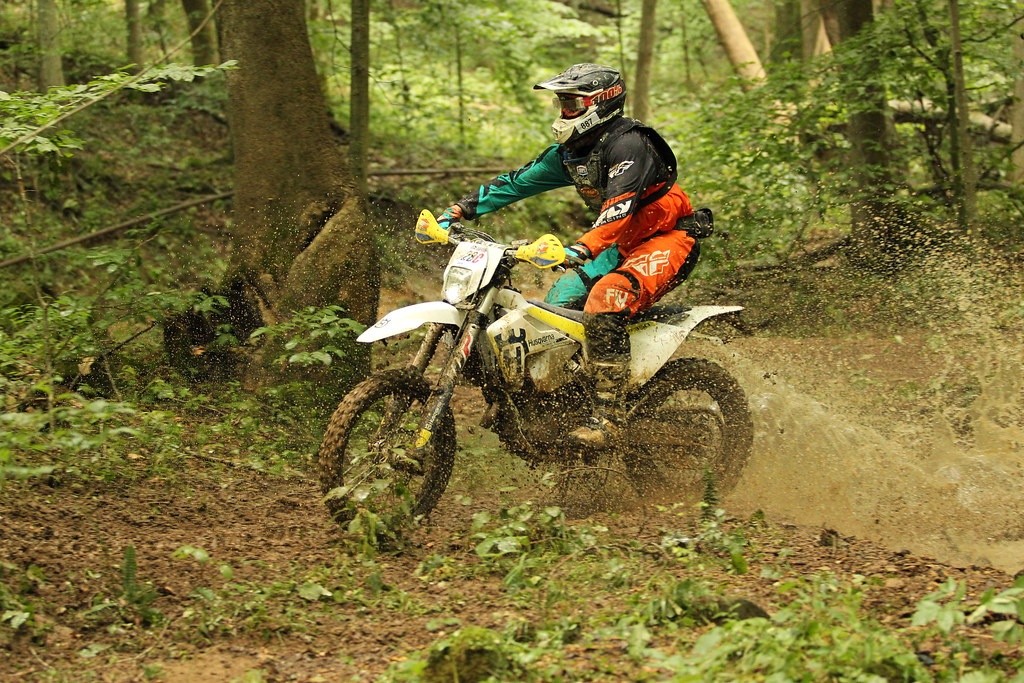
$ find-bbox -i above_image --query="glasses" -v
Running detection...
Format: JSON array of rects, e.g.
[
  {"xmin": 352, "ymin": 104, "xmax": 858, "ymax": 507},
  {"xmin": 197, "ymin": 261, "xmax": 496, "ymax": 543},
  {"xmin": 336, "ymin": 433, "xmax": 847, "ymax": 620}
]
[{"xmin": 552, "ymin": 94, "xmax": 586, "ymax": 114}]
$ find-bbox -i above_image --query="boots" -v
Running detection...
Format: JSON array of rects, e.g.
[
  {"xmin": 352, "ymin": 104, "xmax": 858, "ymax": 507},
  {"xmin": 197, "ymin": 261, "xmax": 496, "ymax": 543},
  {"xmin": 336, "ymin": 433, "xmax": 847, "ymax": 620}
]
[{"xmin": 570, "ymin": 361, "xmax": 627, "ymax": 448}]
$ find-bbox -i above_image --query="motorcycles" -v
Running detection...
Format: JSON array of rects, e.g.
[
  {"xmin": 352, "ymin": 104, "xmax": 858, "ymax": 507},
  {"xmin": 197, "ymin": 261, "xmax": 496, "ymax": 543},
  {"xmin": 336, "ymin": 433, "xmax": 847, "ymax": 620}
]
[{"xmin": 316, "ymin": 210, "xmax": 756, "ymax": 552}]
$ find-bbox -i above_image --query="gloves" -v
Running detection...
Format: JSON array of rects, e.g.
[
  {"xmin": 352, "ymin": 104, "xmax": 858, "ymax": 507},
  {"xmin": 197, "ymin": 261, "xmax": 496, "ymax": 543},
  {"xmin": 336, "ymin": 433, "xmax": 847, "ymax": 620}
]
[
  {"xmin": 436, "ymin": 207, "xmax": 462, "ymax": 230},
  {"xmin": 542, "ymin": 243, "xmax": 590, "ymax": 272}
]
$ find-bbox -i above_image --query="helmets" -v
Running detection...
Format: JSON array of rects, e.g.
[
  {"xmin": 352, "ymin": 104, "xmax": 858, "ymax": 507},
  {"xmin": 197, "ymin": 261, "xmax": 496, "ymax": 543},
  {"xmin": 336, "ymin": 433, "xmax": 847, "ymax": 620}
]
[{"xmin": 533, "ymin": 64, "xmax": 627, "ymax": 144}]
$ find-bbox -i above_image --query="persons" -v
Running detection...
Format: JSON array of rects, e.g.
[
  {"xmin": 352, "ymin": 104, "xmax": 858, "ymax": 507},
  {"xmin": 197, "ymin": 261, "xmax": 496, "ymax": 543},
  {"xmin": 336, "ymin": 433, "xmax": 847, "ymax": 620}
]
[{"xmin": 438, "ymin": 64, "xmax": 700, "ymax": 450}]
